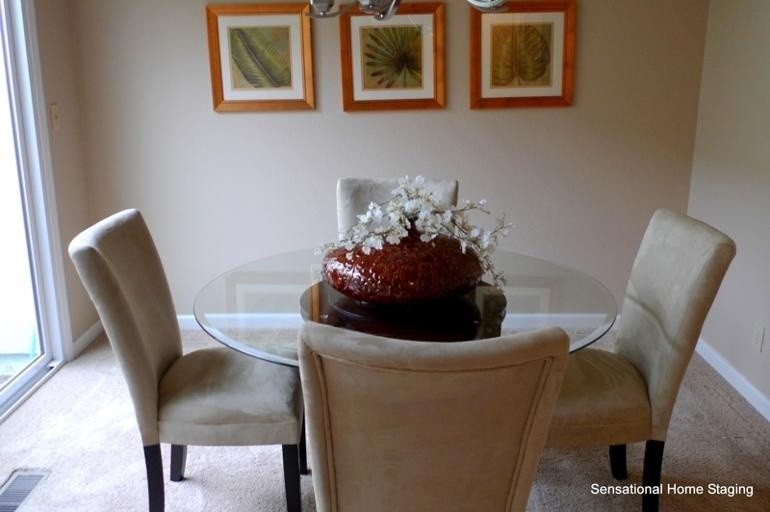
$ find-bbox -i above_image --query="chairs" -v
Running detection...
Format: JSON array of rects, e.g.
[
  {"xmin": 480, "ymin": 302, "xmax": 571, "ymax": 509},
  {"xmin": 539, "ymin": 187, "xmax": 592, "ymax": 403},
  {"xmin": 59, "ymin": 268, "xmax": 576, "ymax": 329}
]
[
  {"xmin": 297, "ymin": 320, "xmax": 569, "ymax": 512},
  {"xmin": 542, "ymin": 208, "xmax": 737, "ymax": 510},
  {"xmin": 63, "ymin": 206, "xmax": 310, "ymax": 511},
  {"xmin": 336, "ymin": 176, "xmax": 461, "ymax": 244}
]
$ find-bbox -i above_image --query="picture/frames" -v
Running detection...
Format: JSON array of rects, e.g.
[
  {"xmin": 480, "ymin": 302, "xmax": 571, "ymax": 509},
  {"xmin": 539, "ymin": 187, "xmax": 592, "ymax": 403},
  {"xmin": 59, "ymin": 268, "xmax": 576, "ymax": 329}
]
[
  {"xmin": 469, "ymin": 1, "xmax": 576, "ymax": 109},
  {"xmin": 340, "ymin": 2, "xmax": 445, "ymax": 112},
  {"xmin": 205, "ymin": 3, "xmax": 317, "ymax": 113}
]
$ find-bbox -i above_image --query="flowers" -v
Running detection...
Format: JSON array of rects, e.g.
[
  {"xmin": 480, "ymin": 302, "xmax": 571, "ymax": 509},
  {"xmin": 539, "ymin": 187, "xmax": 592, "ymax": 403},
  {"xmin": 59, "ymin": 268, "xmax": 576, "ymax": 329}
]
[{"xmin": 311, "ymin": 171, "xmax": 513, "ymax": 286}]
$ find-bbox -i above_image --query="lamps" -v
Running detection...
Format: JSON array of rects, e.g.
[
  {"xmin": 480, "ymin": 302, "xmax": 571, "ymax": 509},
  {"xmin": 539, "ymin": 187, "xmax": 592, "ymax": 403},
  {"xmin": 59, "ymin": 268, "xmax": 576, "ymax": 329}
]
[{"xmin": 307, "ymin": 1, "xmax": 508, "ymax": 23}]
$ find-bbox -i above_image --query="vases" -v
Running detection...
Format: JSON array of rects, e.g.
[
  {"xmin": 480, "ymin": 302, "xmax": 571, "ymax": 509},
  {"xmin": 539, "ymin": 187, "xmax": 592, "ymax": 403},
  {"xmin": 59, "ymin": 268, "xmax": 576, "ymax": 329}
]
[{"xmin": 322, "ymin": 218, "xmax": 484, "ymax": 305}]
